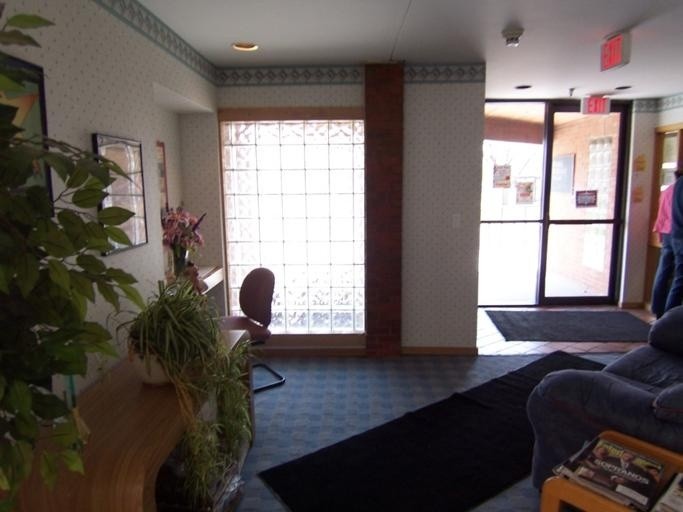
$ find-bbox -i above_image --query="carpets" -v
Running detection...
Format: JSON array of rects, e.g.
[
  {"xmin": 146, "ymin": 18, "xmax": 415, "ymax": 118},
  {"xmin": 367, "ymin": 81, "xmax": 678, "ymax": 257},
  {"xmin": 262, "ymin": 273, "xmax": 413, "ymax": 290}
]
[
  {"xmin": 484, "ymin": 308, "xmax": 651, "ymax": 344},
  {"xmin": 258, "ymin": 348, "xmax": 608, "ymax": 512}
]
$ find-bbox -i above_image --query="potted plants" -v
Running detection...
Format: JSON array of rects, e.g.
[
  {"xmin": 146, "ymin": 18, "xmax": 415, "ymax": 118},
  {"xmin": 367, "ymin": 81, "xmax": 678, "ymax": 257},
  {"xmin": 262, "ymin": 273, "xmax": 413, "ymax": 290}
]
[{"xmin": 122, "ymin": 277, "xmax": 256, "ymax": 512}]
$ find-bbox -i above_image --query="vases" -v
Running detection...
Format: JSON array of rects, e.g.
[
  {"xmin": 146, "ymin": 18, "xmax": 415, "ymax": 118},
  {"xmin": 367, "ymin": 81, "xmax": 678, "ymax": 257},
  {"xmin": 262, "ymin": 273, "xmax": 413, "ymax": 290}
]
[{"xmin": 170, "ymin": 246, "xmax": 187, "ymax": 278}]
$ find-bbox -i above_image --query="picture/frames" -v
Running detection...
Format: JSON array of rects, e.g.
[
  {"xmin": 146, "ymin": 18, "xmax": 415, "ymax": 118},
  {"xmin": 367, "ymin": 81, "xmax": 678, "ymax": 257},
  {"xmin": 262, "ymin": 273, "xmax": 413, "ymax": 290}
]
[{"xmin": 0, "ymin": 53, "xmax": 148, "ymax": 260}]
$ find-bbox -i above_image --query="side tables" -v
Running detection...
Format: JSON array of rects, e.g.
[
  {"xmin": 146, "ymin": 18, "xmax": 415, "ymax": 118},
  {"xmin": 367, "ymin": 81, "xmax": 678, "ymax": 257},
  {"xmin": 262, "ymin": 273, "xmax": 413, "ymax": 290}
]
[{"xmin": 538, "ymin": 429, "xmax": 682, "ymax": 512}]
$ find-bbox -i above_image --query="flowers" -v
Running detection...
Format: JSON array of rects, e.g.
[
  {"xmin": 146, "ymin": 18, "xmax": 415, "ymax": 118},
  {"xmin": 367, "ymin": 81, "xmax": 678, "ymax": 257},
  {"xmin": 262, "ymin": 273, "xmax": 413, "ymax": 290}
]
[{"xmin": 158, "ymin": 202, "xmax": 207, "ymax": 271}]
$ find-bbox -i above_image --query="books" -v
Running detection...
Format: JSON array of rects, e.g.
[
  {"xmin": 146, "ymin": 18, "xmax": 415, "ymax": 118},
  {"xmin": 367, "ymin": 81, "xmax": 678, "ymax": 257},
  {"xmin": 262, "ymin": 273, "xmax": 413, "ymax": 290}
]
[{"xmin": 552, "ymin": 436, "xmax": 683, "ymax": 512}]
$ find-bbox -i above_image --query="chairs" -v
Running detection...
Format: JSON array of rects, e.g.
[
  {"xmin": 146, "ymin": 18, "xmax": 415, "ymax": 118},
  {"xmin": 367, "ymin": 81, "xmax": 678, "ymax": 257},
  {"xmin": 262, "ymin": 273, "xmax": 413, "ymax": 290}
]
[
  {"xmin": 214, "ymin": 268, "xmax": 285, "ymax": 394},
  {"xmin": 526, "ymin": 304, "xmax": 682, "ymax": 490}
]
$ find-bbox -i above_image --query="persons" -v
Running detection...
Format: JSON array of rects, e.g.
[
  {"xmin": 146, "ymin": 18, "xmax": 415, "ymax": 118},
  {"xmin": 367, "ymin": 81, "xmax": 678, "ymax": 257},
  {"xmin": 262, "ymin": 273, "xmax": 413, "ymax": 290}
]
[
  {"xmin": 647, "ymin": 170, "xmax": 683, "ymax": 320},
  {"xmin": 664, "ymin": 175, "xmax": 683, "ymax": 312}
]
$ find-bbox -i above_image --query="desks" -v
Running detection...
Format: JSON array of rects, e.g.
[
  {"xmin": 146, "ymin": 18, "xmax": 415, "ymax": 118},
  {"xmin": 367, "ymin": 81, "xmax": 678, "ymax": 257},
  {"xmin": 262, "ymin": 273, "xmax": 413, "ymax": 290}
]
[{"xmin": 7, "ymin": 327, "xmax": 260, "ymax": 512}]
